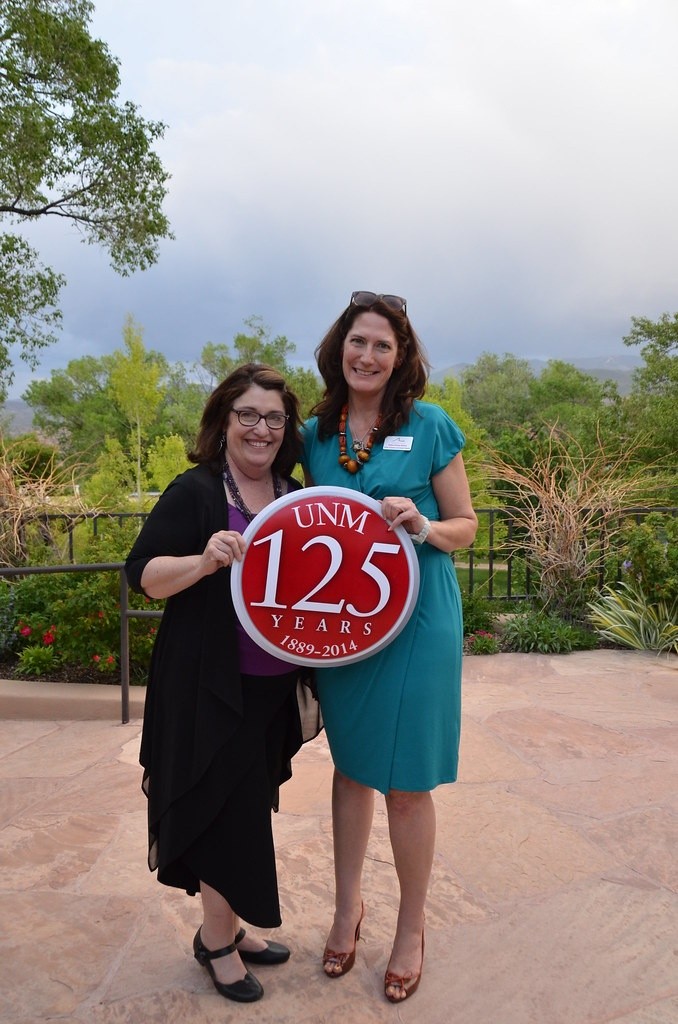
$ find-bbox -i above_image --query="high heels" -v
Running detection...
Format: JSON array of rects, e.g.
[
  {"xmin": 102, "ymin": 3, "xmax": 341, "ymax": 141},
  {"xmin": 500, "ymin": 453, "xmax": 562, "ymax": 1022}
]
[
  {"xmin": 384, "ymin": 912, "xmax": 425, "ymax": 1004},
  {"xmin": 322, "ymin": 898, "xmax": 365, "ymax": 979},
  {"xmin": 234, "ymin": 926, "xmax": 290, "ymax": 965},
  {"xmin": 192, "ymin": 924, "xmax": 264, "ymax": 1003}
]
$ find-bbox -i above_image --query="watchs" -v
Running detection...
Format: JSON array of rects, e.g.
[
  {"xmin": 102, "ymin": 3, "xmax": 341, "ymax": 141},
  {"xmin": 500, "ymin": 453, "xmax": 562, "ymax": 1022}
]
[{"xmin": 407, "ymin": 515, "xmax": 430, "ymax": 544}]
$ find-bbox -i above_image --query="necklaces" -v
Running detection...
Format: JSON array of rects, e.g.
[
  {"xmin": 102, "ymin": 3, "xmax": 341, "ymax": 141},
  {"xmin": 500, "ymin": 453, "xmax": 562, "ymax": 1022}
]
[
  {"xmin": 348, "ymin": 412, "xmax": 377, "ymax": 453},
  {"xmin": 338, "ymin": 404, "xmax": 381, "ymax": 473},
  {"xmin": 222, "ymin": 458, "xmax": 283, "ymax": 523}
]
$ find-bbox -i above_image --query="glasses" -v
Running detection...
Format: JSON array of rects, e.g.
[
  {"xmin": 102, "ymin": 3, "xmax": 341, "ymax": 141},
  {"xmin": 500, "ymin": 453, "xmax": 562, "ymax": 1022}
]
[
  {"xmin": 231, "ymin": 407, "xmax": 290, "ymax": 430},
  {"xmin": 350, "ymin": 290, "xmax": 407, "ymax": 315}
]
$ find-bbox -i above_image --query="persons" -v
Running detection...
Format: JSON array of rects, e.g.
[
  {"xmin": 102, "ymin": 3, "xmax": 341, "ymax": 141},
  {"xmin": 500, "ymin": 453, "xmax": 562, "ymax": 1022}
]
[
  {"xmin": 123, "ymin": 364, "xmax": 324, "ymax": 1003},
  {"xmin": 294, "ymin": 290, "xmax": 479, "ymax": 1003}
]
